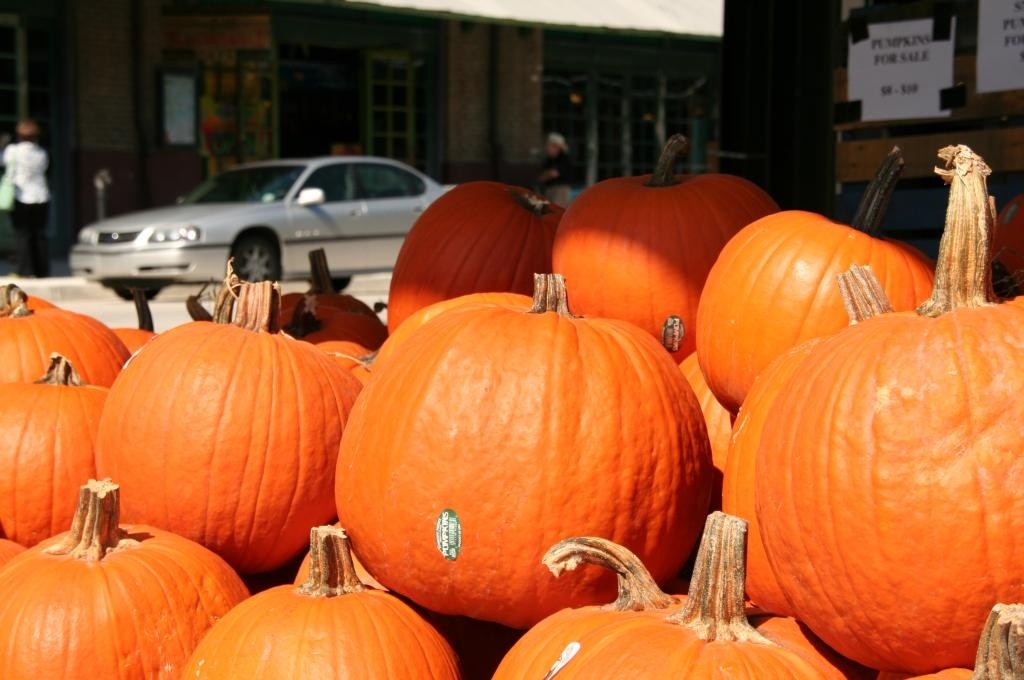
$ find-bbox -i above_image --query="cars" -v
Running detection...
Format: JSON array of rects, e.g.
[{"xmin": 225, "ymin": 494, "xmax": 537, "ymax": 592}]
[{"xmin": 70, "ymin": 155, "xmax": 463, "ymax": 299}]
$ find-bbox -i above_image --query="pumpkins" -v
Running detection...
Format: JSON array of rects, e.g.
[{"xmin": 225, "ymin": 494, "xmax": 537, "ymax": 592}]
[{"xmin": 0, "ymin": 132, "xmax": 1024, "ymax": 679}]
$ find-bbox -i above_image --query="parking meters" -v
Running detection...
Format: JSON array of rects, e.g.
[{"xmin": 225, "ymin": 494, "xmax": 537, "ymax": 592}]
[{"xmin": 95, "ymin": 167, "xmax": 112, "ymax": 220}]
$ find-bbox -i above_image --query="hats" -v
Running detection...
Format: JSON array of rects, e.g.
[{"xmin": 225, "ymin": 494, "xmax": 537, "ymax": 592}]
[
  {"xmin": 549, "ymin": 133, "xmax": 568, "ymax": 151},
  {"xmin": 18, "ymin": 122, "xmax": 37, "ymax": 135}
]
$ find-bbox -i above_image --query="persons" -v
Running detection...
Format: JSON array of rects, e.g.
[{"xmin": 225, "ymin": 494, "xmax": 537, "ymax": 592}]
[
  {"xmin": 1, "ymin": 121, "xmax": 54, "ymax": 279},
  {"xmin": 539, "ymin": 132, "xmax": 582, "ymax": 208}
]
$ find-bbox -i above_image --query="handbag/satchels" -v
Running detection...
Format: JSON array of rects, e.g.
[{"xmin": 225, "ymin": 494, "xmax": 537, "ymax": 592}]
[{"xmin": 0, "ymin": 145, "xmax": 15, "ymax": 210}]
